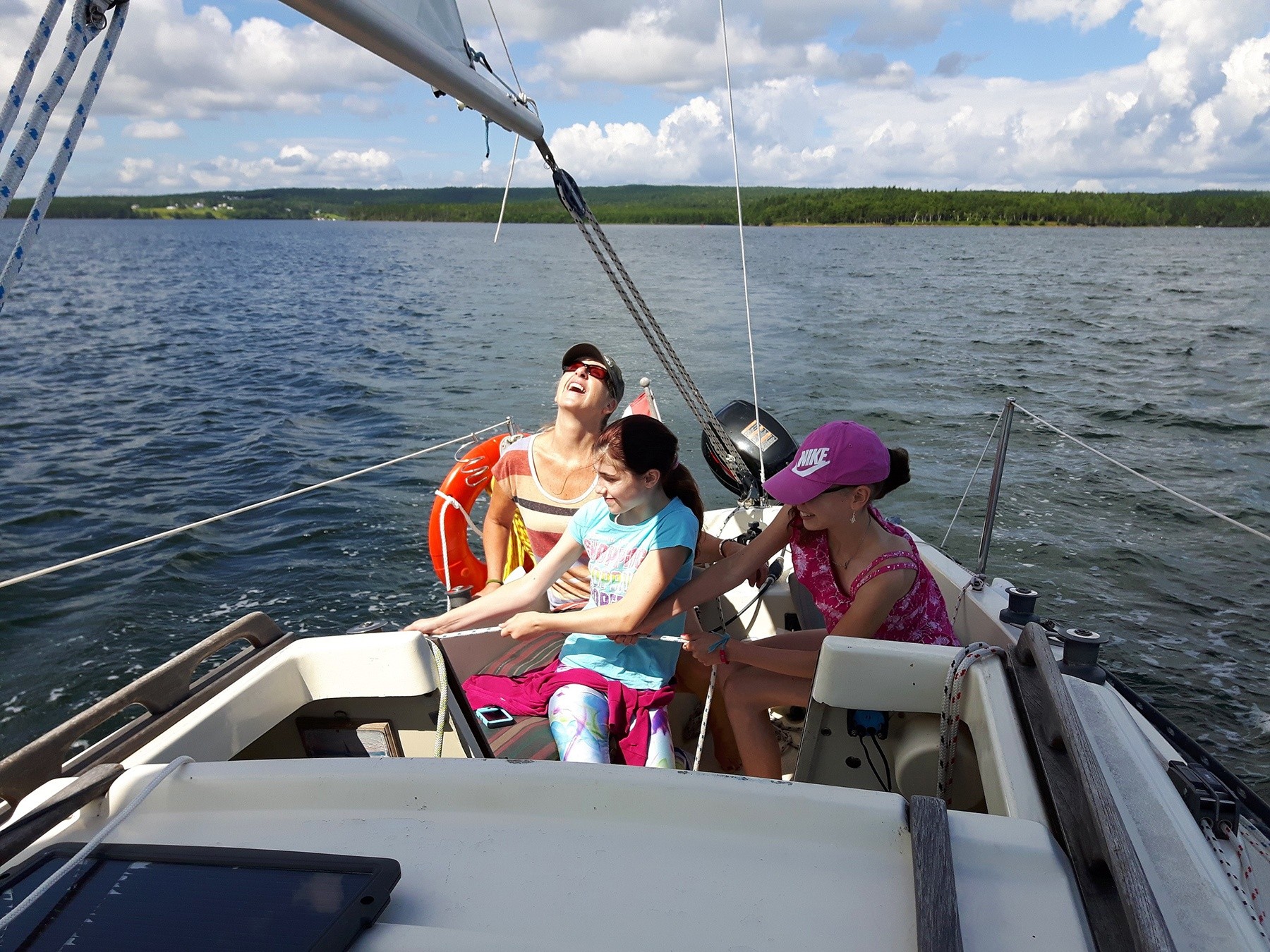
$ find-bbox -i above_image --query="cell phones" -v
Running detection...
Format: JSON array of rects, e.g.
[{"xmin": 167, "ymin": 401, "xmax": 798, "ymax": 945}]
[{"xmin": 476, "ymin": 707, "xmax": 514, "ymax": 728}]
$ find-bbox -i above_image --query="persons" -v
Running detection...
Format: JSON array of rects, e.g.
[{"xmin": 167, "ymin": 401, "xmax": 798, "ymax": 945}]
[
  {"xmin": 608, "ymin": 421, "xmax": 961, "ymax": 783},
  {"xmin": 472, "ymin": 342, "xmax": 768, "ymax": 776},
  {"xmin": 405, "ymin": 414, "xmax": 703, "ymax": 769}
]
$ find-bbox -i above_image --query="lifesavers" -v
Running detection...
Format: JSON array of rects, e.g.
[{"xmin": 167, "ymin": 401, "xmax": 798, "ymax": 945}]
[{"xmin": 429, "ymin": 432, "xmax": 532, "ymax": 601}]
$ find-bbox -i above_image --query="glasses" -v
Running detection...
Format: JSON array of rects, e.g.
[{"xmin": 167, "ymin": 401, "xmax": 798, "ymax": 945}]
[{"xmin": 563, "ymin": 360, "xmax": 615, "ymax": 399}]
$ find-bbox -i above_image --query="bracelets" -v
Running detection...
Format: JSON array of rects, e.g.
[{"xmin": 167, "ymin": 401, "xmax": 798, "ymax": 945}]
[
  {"xmin": 707, "ymin": 631, "xmax": 732, "ymax": 664},
  {"xmin": 485, "ymin": 579, "xmax": 504, "ymax": 585},
  {"xmin": 718, "ymin": 538, "xmax": 736, "ymax": 558}
]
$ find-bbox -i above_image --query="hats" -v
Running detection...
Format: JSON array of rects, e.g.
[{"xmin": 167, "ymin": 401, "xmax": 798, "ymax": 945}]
[
  {"xmin": 562, "ymin": 342, "xmax": 624, "ymax": 404},
  {"xmin": 761, "ymin": 420, "xmax": 890, "ymax": 505}
]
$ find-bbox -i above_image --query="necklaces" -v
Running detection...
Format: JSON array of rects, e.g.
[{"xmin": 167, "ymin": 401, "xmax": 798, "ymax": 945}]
[{"xmin": 831, "ymin": 516, "xmax": 870, "ymax": 569}]
